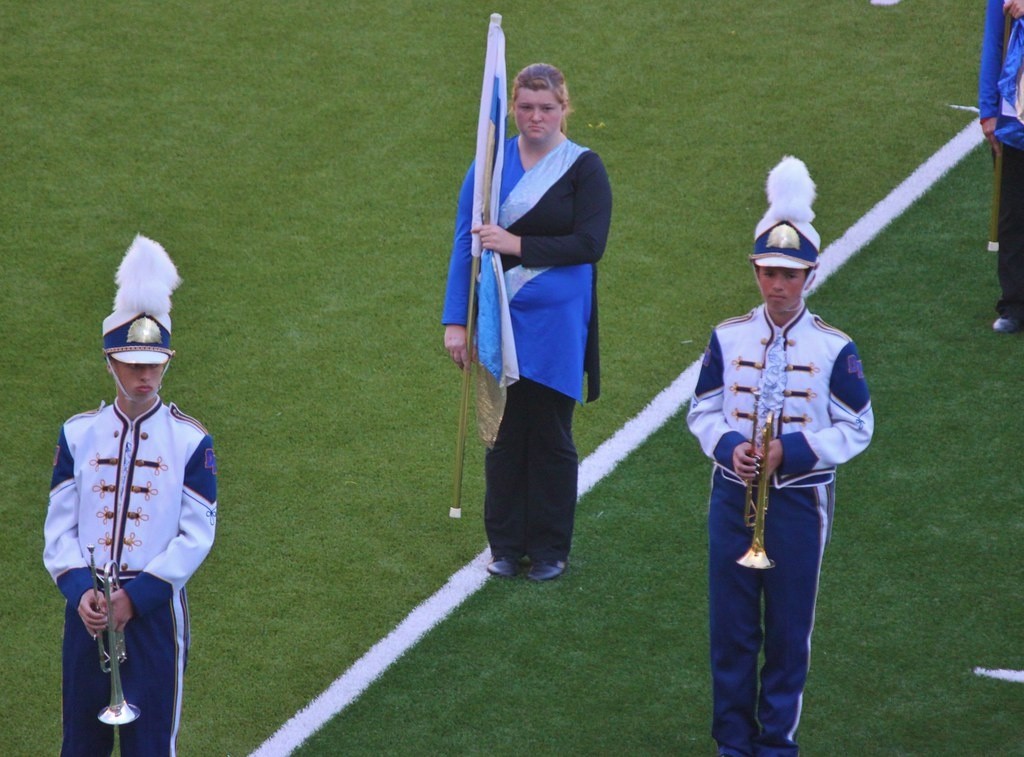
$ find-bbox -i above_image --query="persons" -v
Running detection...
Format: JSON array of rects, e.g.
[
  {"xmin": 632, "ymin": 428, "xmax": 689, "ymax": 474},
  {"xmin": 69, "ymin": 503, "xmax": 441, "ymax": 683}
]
[
  {"xmin": 39, "ymin": 232, "xmax": 221, "ymax": 757},
  {"xmin": 442, "ymin": 61, "xmax": 615, "ymax": 582},
  {"xmin": 969, "ymin": 1, "xmax": 1024, "ymax": 331},
  {"xmin": 685, "ymin": 154, "xmax": 877, "ymax": 757}
]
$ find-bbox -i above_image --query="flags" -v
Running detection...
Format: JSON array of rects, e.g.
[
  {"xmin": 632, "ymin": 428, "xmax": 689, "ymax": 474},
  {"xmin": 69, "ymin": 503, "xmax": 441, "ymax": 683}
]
[{"xmin": 472, "ymin": 19, "xmax": 519, "ymax": 449}]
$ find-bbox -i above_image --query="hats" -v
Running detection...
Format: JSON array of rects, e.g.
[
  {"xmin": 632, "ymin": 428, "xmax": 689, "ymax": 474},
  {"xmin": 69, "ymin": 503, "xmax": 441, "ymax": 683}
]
[
  {"xmin": 749, "ymin": 209, "xmax": 823, "ymax": 269},
  {"xmin": 101, "ymin": 310, "xmax": 174, "ymax": 364}
]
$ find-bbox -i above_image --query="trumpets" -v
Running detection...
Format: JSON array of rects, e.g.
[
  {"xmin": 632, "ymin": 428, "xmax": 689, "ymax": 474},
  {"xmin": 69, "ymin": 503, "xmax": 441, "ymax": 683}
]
[
  {"xmin": 736, "ymin": 389, "xmax": 775, "ymax": 569},
  {"xmin": 86, "ymin": 543, "xmax": 141, "ymax": 726}
]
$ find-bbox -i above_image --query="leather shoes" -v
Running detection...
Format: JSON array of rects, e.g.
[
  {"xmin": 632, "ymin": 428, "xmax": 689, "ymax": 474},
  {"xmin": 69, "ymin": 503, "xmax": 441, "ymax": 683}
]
[
  {"xmin": 528, "ymin": 555, "xmax": 566, "ymax": 583},
  {"xmin": 485, "ymin": 554, "xmax": 522, "ymax": 578}
]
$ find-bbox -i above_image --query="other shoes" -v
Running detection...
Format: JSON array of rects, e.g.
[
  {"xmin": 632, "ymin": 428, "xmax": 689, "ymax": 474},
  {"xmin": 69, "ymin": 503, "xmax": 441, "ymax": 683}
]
[{"xmin": 994, "ymin": 312, "xmax": 1019, "ymax": 336}]
